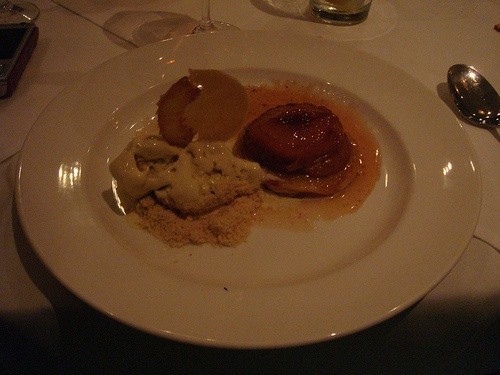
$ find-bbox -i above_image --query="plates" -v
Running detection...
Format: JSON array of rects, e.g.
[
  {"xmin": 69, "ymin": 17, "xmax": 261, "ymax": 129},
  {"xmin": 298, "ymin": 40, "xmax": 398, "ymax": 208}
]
[{"xmin": 15, "ymin": 28, "xmax": 482, "ymax": 350}]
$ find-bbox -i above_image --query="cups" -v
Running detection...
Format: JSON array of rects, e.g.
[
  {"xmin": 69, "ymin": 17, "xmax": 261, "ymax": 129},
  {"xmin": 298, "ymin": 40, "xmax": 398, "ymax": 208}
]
[{"xmin": 309, "ymin": 0, "xmax": 372, "ymax": 27}]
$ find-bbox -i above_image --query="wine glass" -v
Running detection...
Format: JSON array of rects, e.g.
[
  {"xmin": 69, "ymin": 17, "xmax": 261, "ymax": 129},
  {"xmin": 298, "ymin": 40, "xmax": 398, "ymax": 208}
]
[
  {"xmin": 0, "ymin": 0, "xmax": 39, "ymax": 24},
  {"xmin": 169, "ymin": 0, "xmax": 240, "ymax": 38}
]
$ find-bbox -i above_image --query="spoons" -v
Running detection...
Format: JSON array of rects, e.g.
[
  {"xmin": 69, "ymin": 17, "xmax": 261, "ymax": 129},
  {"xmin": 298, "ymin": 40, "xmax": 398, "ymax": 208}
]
[{"xmin": 447, "ymin": 64, "xmax": 500, "ymax": 128}]
